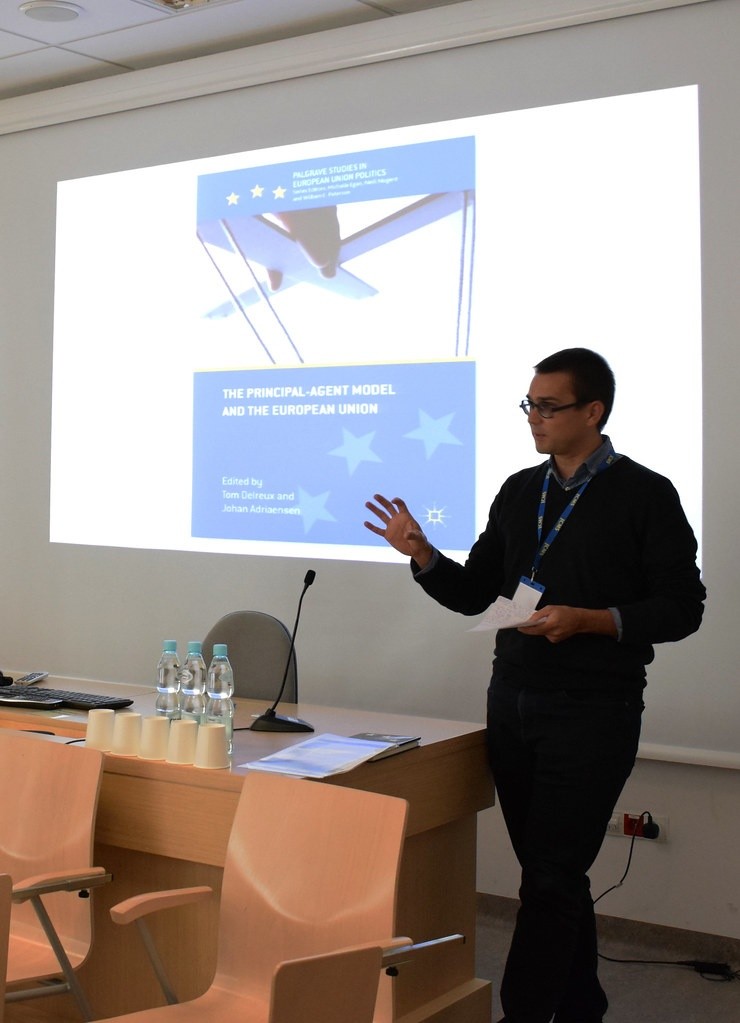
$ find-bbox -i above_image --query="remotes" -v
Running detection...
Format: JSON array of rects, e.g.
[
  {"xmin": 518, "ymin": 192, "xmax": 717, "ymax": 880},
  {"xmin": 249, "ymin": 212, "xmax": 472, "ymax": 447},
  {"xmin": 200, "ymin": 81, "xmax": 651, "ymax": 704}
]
[
  {"xmin": 0, "ymin": 693, "xmax": 63, "ymax": 710},
  {"xmin": 14, "ymin": 672, "xmax": 49, "ymax": 687}
]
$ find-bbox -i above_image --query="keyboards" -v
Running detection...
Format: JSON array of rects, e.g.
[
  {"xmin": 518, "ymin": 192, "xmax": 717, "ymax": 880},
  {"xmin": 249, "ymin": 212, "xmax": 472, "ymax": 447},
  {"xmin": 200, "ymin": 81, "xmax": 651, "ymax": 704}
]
[{"xmin": 0, "ymin": 685, "xmax": 134, "ymax": 712}]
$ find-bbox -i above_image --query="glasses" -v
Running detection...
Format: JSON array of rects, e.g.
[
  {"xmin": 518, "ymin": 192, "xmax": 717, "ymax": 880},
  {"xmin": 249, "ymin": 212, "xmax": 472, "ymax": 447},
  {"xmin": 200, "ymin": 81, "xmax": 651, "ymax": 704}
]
[{"xmin": 519, "ymin": 399, "xmax": 593, "ymax": 419}]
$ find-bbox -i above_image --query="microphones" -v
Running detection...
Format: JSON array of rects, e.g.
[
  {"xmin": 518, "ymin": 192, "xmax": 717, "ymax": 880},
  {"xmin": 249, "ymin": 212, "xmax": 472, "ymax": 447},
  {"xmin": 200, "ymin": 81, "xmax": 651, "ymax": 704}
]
[{"xmin": 250, "ymin": 569, "xmax": 316, "ymax": 733}]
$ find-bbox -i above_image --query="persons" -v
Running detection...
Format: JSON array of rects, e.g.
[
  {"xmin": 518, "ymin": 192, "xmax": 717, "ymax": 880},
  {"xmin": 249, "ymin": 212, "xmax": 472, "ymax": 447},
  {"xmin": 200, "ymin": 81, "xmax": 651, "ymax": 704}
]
[{"xmin": 363, "ymin": 347, "xmax": 707, "ymax": 1023}]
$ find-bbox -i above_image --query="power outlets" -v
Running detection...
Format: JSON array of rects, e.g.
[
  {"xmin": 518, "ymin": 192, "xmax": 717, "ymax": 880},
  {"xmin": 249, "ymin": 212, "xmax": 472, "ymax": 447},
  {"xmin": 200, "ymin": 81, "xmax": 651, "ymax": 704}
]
[{"xmin": 605, "ymin": 809, "xmax": 670, "ymax": 844}]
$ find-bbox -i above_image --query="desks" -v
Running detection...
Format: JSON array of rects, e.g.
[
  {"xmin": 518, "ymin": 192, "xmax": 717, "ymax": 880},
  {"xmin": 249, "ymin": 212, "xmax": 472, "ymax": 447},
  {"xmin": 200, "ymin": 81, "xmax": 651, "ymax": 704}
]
[{"xmin": 0, "ymin": 671, "xmax": 495, "ymax": 1023}]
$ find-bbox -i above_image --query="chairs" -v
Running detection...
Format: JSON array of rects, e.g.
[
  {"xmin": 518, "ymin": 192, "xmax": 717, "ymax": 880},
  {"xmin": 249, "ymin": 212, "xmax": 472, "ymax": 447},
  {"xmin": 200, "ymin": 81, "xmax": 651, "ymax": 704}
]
[
  {"xmin": 0, "ymin": 727, "xmax": 115, "ymax": 1023},
  {"xmin": 201, "ymin": 609, "xmax": 299, "ymax": 703},
  {"xmin": 90, "ymin": 770, "xmax": 468, "ymax": 1023}
]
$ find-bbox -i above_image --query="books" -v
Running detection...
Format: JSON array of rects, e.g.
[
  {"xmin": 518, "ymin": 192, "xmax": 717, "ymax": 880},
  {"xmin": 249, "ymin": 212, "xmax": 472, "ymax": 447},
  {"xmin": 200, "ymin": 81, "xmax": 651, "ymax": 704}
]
[{"xmin": 237, "ymin": 730, "xmax": 422, "ymax": 779}]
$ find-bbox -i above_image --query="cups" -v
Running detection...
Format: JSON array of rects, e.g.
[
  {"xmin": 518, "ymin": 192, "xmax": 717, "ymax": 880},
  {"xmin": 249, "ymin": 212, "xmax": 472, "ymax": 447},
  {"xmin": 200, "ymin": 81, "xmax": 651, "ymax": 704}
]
[
  {"xmin": 85, "ymin": 709, "xmax": 114, "ymax": 751},
  {"xmin": 138, "ymin": 716, "xmax": 169, "ymax": 761},
  {"xmin": 194, "ymin": 723, "xmax": 229, "ymax": 769},
  {"xmin": 167, "ymin": 719, "xmax": 198, "ymax": 764},
  {"xmin": 111, "ymin": 713, "xmax": 142, "ymax": 758}
]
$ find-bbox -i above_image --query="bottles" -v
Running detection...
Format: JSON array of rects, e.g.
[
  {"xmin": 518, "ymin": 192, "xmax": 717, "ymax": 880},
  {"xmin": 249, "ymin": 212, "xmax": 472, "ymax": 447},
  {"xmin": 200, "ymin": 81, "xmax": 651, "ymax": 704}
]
[
  {"xmin": 204, "ymin": 644, "xmax": 234, "ymax": 756},
  {"xmin": 154, "ymin": 640, "xmax": 182, "ymax": 723},
  {"xmin": 180, "ymin": 641, "xmax": 207, "ymax": 725}
]
